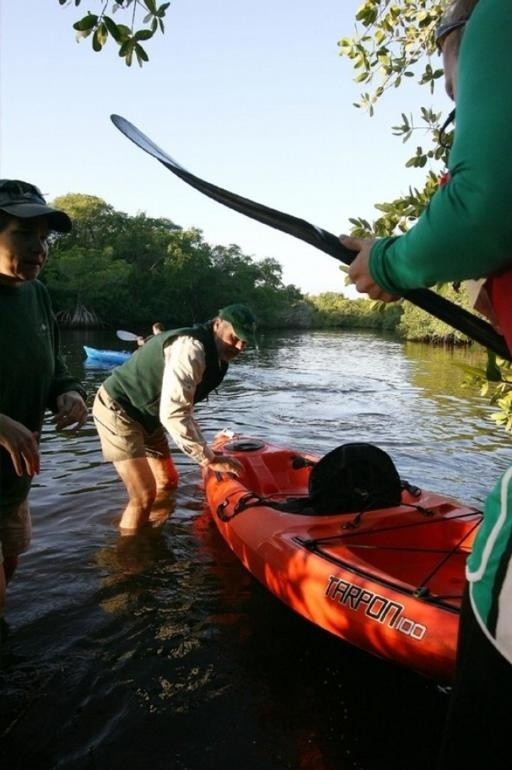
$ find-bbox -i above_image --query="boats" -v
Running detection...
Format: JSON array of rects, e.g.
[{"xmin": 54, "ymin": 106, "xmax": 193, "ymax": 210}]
[
  {"xmin": 82, "ymin": 346, "xmax": 133, "ymax": 366},
  {"xmin": 204, "ymin": 424, "xmax": 488, "ymax": 688}
]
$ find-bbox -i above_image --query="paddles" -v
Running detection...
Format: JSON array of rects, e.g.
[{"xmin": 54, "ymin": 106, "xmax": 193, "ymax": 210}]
[
  {"xmin": 116, "ymin": 330, "xmax": 140, "ymax": 343},
  {"xmin": 111, "ymin": 116, "xmax": 511, "ymax": 362}
]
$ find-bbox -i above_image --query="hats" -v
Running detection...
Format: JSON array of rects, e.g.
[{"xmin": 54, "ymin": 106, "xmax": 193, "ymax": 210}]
[
  {"xmin": 219, "ymin": 304, "xmax": 256, "ymax": 347},
  {"xmin": 1, "ymin": 179, "xmax": 72, "ymax": 233}
]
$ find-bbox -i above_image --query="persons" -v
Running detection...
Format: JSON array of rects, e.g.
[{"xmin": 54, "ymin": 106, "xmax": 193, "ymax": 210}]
[
  {"xmin": 435, "ymin": 0, "xmax": 476, "ymax": 104},
  {"xmin": 0, "ymin": 177, "xmax": 89, "ymax": 633},
  {"xmin": 131, "ymin": 320, "xmax": 164, "ymax": 356},
  {"xmin": 89, "ymin": 302, "xmax": 260, "ymax": 531},
  {"xmin": 335, "ymin": 0, "xmax": 512, "ymax": 770}
]
